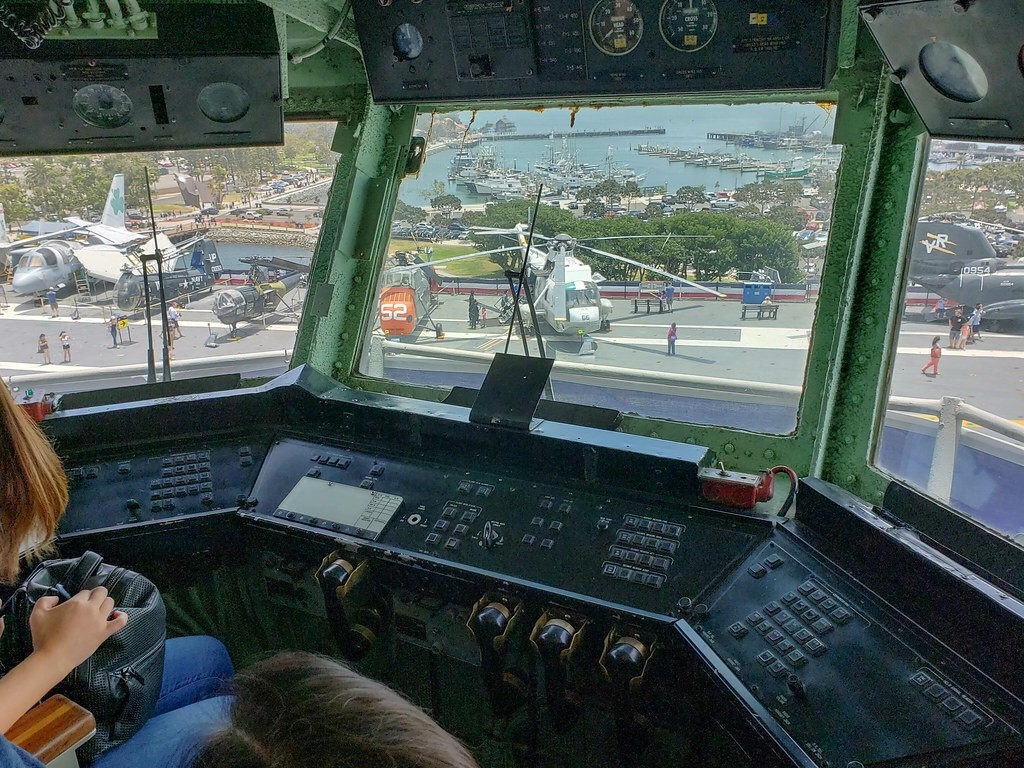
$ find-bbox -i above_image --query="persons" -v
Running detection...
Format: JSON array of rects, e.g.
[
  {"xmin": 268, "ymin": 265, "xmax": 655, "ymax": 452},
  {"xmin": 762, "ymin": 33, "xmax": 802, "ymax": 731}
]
[
  {"xmin": 667, "ymin": 322, "xmax": 677, "ymax": 355},
  {"xmin": 0, "ymin": 374, "xmax": 478, "ymax": 768},
  {"xmin": 39, "ymin": 334, "xmax": 51, "ymax": 364},
  {"xmin": 935, "ymin": 298, "xmax": 945, "ymax": 319},
  {"xmin": 108, "ymin": 316, "xmax": 119, "ymax": 348},
  {"xmin": 761, "ymin": 296, "xmax": 772, "ymax": 318},
  {"xmin": 661, "ymin": 282, "xmax": 674, "ymax": 312},
  {"xmin": 921, "ymin": 336, "xmax": 941, "ymax": 375},
  {"xmin": 160, "ymin": 302, "xmax": 184, "ymax": 361},
  {"xmin": 948, "ymin": 303, "xmax": 986, "ymax": 351},
  {"xmin": 469, "ymin": 291, "xmax": 486, "ymax": 329},
  {"xmin": 46, "ymin": 287, "xmax": 59, "ymax": 318},
  {"xmin": 59, "ymin": 331, "xmax": 71, "ymax": 363},
  {"xmin": 501, "ymin": 290, "xmax": 508, "ymax": 316}
]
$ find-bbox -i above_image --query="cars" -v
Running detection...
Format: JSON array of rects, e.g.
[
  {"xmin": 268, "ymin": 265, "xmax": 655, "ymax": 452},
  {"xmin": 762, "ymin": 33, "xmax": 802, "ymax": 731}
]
[
  {"xmin": 568, "ymin": 201, "xmax": 578, "ymax": 209},
  {"xmin": 125, "ymin": 207, "xmax": 157, "ymax": 230},
  {"xmin": 716, "ymin": 192, "xmax": 730, "ymax": 200},
  {"xmin": 549, "ymin": 201, "xmax": 560, "ymax": 208},
  {"xmin": 231, "ymin": 208, "xmax": 249, "ymax": 215},
  {"xmin": 822, "ymin": 222, "xmax": 830, "ymax": 231},
  {"xmin": 705, "ymin": 192, "xmax": 715, "ymax": 201},
  {"xmin": 799, "ymin": 231, "xmax": 816, "ymax": 242},
  {"xmin": 200, "ymin": 207, "xmax": 219, "ymax": 215},
  {"xmin": 803, "ymin": 262, "xmax": 819, "ymax": 274},
  {"xmin": 815, "ymin": 211, "xmax": 830, "ymax": 220},
  {"xmin": 799, "ymin": 188, "xmax": 832, "ymax": 209},
  {"xmin": 254, "ymin": 164, "xmax": 309, "ymax": 194},
  {"xmin": 594, "ymin": 194, "xmax": 691, "ymax": 219},
  {"xmin": 946, "ymin": 180, "xmax": 1017, "ymax": 214},
  {"xmin": 744, "ymin": 188, "xmax": 786, "ymax": 201},
  {"xmin": 264, "ymin": 208, "xmax": 273, "ymax": 213},
  {"xmin": 805, "ymin": 222, "xmax": 819, "ymax": 231},
  {"xmin": 151, "ymin": 156, "xmax": 249, "ymax": 194},
  {"xmin": 388, "ymin": 219, "xmax": 469, "ymax": 241}
]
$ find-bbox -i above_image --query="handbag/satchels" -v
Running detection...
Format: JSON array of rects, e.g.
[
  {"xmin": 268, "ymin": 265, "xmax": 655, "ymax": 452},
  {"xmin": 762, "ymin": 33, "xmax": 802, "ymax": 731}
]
[
  {"xmin": 1, "ymin": 551, "xmax": 167, "ymax": 764},
  {"xmin": 931, "ymin": 348, "xmax": 941, "ymax": 358},
  {"xmin": 670, "ymin": 335, "xmax": 677, "ymax": 340}
]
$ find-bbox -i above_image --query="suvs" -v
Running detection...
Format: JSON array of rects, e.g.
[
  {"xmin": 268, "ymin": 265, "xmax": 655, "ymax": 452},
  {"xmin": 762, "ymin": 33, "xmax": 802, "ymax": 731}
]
[{"xmin": 277, "ymin": 208, "xmax": 293, "ymax": 216}]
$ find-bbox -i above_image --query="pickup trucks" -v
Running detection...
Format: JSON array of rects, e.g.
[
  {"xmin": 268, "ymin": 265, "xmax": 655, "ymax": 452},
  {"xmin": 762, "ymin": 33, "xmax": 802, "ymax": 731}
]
[
  {"xmin": 239, "ymin": 210, "xmax": 263, "ymax": 220},
  {"xmin": 710, "ymin": 198, "xmax": 738, "ymax": 209}
]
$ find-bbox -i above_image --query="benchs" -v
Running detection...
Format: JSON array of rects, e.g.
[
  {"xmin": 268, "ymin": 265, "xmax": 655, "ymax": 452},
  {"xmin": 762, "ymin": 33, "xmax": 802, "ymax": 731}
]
[
  {"xmin": 631, "ymin": 299, "xmax": 665, "ymax": 314},
  {"xmin": 740, "ymin": 304, "xmax": 779, "ymax": 320}
]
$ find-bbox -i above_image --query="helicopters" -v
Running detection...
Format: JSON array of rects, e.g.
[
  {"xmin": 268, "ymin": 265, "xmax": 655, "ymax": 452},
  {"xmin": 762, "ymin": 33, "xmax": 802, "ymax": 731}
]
[{"xmin": 371, "ymin": 221, "xmax": 729, "ymax": 337}]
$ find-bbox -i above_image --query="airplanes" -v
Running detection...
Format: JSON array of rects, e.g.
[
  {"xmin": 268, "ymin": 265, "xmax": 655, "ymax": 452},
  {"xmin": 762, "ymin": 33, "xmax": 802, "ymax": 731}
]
[
  {"xmin": 212, "ymin": 254, "xmax": 317, "ymax": 338},
  {"xmin": 907, "ymin": 221, "xmax": 1024, "ymax": 332},
  {"xmin": 0, "ymin": 174, "xmax": 180, "ymax": 294},
  {"xmin": 111, "ymin": 232, "xmax": 225, "ymax": 312}
]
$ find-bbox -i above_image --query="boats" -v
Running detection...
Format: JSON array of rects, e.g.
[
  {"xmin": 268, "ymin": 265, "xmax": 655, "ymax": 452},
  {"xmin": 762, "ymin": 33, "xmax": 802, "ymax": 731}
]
[
  {"xmin": 447, "ymin": 128, "xmax": 647, "ymax": 200},
  {"xmin": 638, "ymin": 107, "xmax": 843, "ymax": 181}
]
